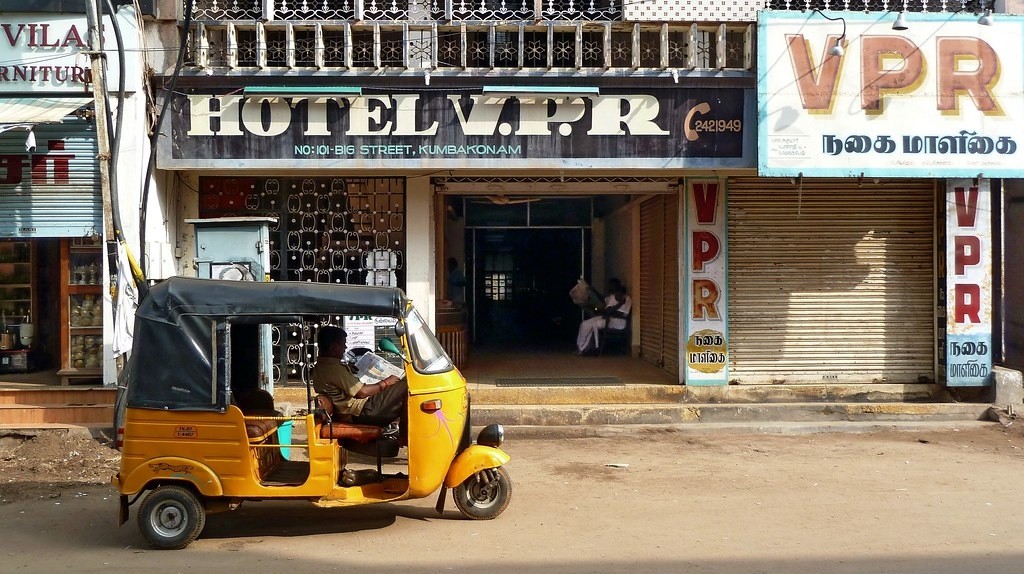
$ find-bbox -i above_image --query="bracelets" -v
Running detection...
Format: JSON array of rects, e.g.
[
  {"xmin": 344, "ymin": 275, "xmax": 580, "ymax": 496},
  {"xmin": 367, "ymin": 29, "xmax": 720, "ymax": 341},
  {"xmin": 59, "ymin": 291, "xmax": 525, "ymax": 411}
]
[{"xmin": 381, "ymin": 380, "xmax": 387, "ymax": 387}]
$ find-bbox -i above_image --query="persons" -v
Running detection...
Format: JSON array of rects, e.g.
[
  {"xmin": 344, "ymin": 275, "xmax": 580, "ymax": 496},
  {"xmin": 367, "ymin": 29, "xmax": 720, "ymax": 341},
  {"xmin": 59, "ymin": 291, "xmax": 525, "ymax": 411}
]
[
  {"xmin": 314, "ymin": 324, "xmax": 408, "ymax": 448},
  {"xmin": 576, "ymin": 277, "xmax": 632, "ymax": 355}
]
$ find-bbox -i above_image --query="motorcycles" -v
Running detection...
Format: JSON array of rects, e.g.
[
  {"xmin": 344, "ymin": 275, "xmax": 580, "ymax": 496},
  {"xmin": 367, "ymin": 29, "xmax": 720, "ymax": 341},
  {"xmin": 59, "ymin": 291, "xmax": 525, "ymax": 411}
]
[{"xmin": 110, "ymin": 276, "xmax": 512, "ymax": 552}]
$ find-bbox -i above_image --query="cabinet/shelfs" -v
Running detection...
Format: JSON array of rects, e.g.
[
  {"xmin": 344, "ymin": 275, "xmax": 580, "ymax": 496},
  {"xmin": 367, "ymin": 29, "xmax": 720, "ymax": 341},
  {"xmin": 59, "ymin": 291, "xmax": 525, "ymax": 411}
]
[
  {"xmin": 0, "ymin": 238, "xmax": 37, "ymax": 374},
  {"xmin": 56, "ymin": 238, "xmax": 119, "ymax": 386}
]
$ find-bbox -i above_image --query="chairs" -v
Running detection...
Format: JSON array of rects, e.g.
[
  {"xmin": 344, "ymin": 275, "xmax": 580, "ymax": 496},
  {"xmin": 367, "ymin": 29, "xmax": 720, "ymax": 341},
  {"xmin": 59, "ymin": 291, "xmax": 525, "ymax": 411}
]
[{"xmin": 595, "ymin": 307, "xmax": 632, "ymax": 358}]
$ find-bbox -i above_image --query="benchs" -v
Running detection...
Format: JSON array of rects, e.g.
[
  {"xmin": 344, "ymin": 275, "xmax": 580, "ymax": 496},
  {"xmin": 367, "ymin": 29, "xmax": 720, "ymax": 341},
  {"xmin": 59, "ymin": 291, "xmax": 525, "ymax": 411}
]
[
  {"xmin": 233, "ymin": 386, "xmax": 285, "ymax": 443},
  {"xmin": 314, "ymin": 394, "xmax": 399, "ymax": 480}
]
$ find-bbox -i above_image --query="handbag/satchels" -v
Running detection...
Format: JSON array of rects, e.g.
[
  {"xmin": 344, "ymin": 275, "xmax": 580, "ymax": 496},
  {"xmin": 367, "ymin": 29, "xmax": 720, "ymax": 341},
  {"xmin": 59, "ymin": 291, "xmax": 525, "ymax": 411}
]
[{"xmin": 571, "ymin": 280, "xmax": 591, "ymax": 305}]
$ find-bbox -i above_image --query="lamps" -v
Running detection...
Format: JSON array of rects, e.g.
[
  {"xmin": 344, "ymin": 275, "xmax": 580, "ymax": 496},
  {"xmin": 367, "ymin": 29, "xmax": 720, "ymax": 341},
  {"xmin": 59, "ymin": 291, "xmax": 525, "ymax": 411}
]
[
  {"xmin": 482, "ymin": 86, "xmax": 600, "ymax": 98},
  {"xmin": 891, "ymin": 0, "xmax": 908, "ymax": 31},
  {"xmin": 814, "ymin": 5, "xmax": 847, "ymax": 58},
  {"xmin": 432, "ymin": 184, "xmax": 447, "ymax": 194},
  {"xmin": 242, "ymin": 87, "xmax": 362, "ymax": 100},
  {"xmin": 792, "ymin": 177, "xmax": 800, "ymax": 185},
  {"xmin": 974, "ymin": 0, "xmax": 997, "ymax": 25}
]
